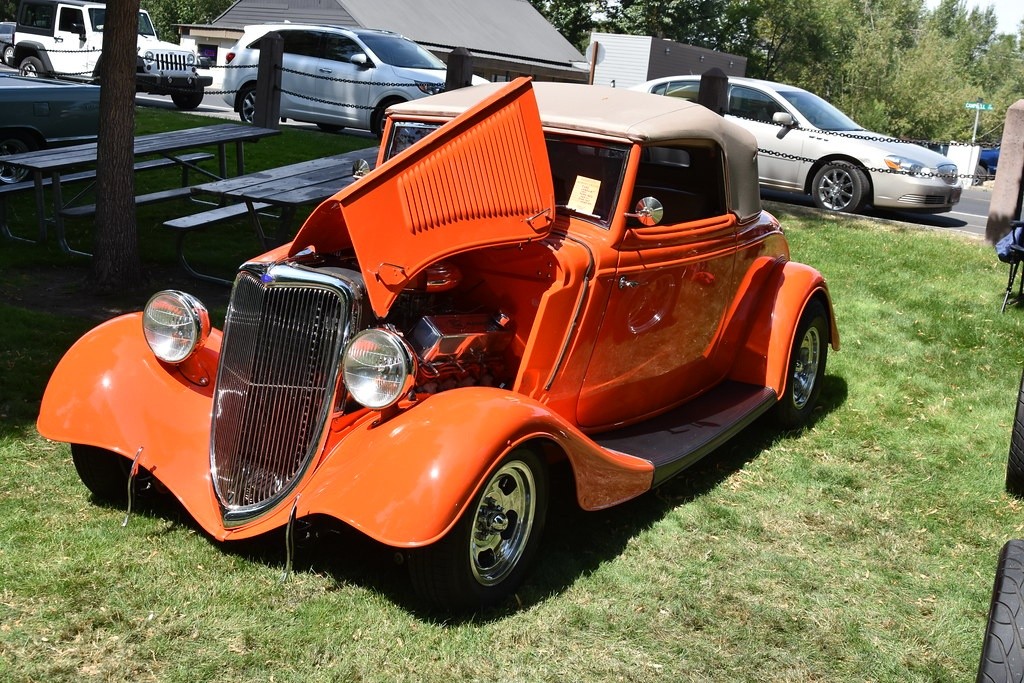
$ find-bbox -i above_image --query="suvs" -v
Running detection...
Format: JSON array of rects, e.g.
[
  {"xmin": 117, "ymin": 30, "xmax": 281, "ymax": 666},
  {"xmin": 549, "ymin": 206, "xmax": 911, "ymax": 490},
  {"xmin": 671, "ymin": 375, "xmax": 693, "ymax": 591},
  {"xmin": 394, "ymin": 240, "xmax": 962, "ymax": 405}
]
[
  {"xmin": 220, "ymin": 21, "xmax": 493, "ymax": 143},
  {"xmin": 12, "ymin": 0, "xmax": 214, "ymax": 111}
]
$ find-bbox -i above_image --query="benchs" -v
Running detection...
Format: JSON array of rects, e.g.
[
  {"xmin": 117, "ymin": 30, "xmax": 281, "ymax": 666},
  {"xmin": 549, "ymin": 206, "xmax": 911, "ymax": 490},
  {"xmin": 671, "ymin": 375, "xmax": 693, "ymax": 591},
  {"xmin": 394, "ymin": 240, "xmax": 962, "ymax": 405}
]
[
  {"xmin": 0, "ymin": 152, "xmax": 214, "ymax": 238},
  {"xmin": 61, "ymin": 178, "xmax": 214, "ymax": 261},
  {"xmin": 162, "ymin": 201, "xmax": 272, "ymax": 277}
]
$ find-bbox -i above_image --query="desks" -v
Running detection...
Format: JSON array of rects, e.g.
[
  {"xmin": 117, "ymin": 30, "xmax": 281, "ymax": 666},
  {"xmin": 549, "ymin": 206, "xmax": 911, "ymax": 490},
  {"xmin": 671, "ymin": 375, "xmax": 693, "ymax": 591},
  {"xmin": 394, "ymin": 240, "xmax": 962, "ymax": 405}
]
[
  {"xmin": 0, "ymin": 125, "xmax": 284, "ymax": 261},
  {"xmin": 176, "ymin": 144, "xmax": 380, "ymax": 285}
]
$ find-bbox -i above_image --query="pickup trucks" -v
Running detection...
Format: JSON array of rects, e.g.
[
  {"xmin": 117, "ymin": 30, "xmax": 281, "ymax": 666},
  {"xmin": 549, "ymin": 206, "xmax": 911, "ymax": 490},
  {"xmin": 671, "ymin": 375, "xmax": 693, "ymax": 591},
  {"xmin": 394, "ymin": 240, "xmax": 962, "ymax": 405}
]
[{"xmin": 0, "ymin": 72, "xmax": 101, "ymax": 186}]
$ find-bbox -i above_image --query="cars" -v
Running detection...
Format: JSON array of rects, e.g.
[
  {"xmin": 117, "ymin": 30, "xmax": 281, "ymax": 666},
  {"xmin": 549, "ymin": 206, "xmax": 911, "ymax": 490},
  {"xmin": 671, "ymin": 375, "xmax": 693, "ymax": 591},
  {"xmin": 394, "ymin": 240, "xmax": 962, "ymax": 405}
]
[
  {"xmin": 0, "ymin": 22, "xmax": 19, "ymax": 67},
  {"xmin": 970, "ymin": 145, "xmax": 1001, "ymax": 187},
  {"xmin": 621, "ymin": 74, "xmax": 962, "ymax": 215},
  {"xmin": 36, "ymin": 78, "xmax": 843, "ymax": 622}
]
